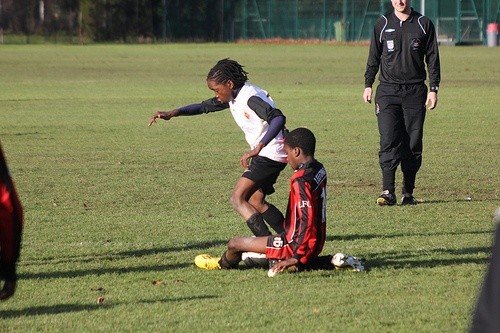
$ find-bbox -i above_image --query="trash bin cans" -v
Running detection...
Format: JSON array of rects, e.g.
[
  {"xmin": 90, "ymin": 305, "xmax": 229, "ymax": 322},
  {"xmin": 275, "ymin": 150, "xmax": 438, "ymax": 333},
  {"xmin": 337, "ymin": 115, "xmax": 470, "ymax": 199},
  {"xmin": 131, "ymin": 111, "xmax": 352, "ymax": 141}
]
[{"xmin": 486, "ymin": 23, "xmax": 500, "ymax": 47}]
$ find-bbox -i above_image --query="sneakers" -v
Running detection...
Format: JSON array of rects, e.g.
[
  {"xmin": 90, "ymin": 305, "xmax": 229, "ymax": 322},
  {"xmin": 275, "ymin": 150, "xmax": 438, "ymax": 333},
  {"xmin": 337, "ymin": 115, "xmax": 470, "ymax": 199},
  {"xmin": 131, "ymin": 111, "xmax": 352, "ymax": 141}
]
[
  {"xmin": 401, "ymin": 193, "xmax": 414, "ymax": 205},
  {"xmin": 331, "ymin": 253, "xmax": 366, "ymax": 270},
  {"xmin": 376, "ymin": 190, "xmax": 397, "ymax": 205},
  {"xmin": 194, "ymin": 254, "xmax": 222, "ymax": 270}
]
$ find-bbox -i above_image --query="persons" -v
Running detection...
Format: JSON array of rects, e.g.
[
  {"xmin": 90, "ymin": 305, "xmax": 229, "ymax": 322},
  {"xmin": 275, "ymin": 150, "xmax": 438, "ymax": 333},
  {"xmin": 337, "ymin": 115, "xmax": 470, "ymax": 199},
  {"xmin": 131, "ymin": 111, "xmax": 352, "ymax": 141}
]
[
  {"xmin": 148, "ymin": 57, "xmax": 366, "ymax": 271},
  {"xmin": 0, "ymin": 144, "xmax": 23, "ymax": 300},
  {"xmin": 363, "ymin": 0, "xmax": 440, "ymax": 206}
]
